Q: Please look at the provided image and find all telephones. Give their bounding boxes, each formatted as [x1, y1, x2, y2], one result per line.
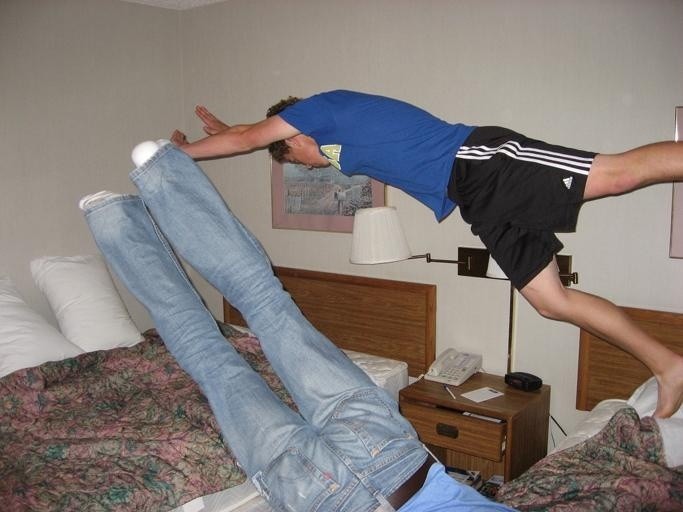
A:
[422, 347, 483, 387]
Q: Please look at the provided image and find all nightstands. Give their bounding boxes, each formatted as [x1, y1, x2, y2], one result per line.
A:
[397, 372, 550, 500]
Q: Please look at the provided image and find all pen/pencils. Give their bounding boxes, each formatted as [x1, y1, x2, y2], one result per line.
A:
[442, 382, 456, 400]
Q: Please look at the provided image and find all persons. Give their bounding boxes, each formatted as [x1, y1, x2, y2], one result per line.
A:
[166, 90, 682, 419]
[76, 135, 525, 511]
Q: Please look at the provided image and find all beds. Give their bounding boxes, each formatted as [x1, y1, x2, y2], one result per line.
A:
[492, 305, 683, 512]
[0, 263, 436, 511]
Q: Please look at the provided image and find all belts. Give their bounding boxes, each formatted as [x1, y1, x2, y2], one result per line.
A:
[374, 454, 435, 511]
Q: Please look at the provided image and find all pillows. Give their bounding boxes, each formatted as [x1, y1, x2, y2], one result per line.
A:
[0, 253, 145, 378]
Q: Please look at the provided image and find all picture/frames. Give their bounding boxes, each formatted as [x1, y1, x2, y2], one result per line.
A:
[268, 151, 387, 234]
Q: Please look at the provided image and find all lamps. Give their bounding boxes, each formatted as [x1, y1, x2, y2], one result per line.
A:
[347, 206, 490, 279]
